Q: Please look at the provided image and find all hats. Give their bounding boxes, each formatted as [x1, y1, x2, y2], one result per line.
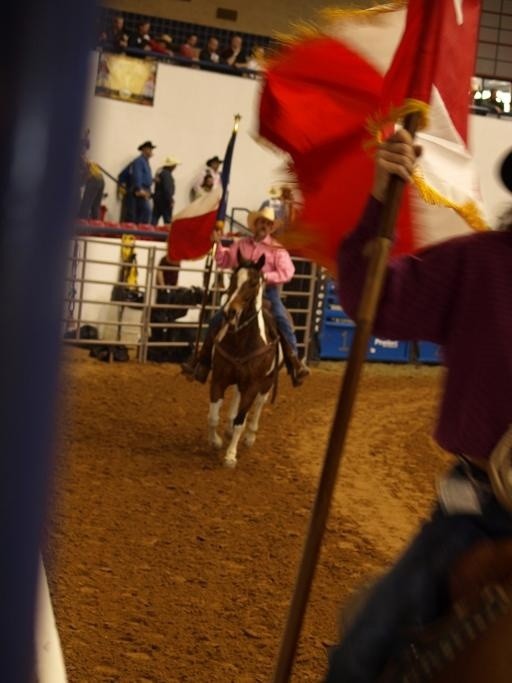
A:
[269, 183, 293, 198]
[248, 207, 283, 233]
[207, 156, 224, 166]
[138, 141, 156, 149]
[163, 156, 182, 167]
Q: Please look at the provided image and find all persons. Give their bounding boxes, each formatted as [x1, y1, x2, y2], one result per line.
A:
[152, 254, 180, 340]
[259, 187, 296, 229]
[480, 89, 504, 114]
[182, 205, 309, 386]
[325, 129, 512, 682]
[117, 142, 179, 226]
[79, 158, 105, 219]
[192, 156, 223, 204]
[99, 14, 268, 80]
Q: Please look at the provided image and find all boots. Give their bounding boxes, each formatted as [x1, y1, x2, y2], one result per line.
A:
[288, 355, 309, 386]
[182, 357, 209, 383]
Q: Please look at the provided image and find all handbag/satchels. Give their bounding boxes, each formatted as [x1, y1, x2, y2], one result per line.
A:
[126, 289, 144, 310]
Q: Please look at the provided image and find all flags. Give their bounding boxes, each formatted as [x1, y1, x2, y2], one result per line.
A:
[258, 0, 501, 272]
[167, 132, 237, 262]
